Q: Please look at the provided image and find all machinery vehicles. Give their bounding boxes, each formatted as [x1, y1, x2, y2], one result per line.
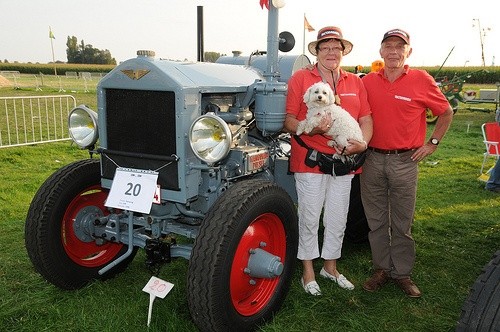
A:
[24, 1, 371, 332]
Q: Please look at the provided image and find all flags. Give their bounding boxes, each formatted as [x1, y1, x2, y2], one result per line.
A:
[50, 31, 55, 39]
[304, 17, 315, 32]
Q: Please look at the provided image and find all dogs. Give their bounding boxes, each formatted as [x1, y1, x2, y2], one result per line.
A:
[296, 81, 364, 149]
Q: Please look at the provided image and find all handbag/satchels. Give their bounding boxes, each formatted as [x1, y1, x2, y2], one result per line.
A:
[292, 130, 366, 176]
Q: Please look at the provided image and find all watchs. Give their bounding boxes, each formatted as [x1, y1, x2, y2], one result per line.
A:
[430, 138, 439, 145]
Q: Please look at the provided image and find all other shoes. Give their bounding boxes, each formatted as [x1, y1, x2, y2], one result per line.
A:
[301, 276, 323, 296]
[320, 267, 354, 291]
[363, 270, 390, 292]
[397, 277, 421, 297]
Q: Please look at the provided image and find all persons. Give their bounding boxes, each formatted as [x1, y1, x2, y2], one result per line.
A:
[359, 29, 454, 298]
[284, 26, 373, 296]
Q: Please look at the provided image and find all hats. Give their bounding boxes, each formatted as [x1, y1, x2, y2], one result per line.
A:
[308, 26, 353, 56]
[382, 29, 409, 46]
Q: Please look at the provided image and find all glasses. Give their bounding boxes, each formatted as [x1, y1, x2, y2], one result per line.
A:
[318, 47, 342, 53]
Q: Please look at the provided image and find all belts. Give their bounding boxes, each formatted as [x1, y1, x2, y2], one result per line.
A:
[368, 146, 408, 156]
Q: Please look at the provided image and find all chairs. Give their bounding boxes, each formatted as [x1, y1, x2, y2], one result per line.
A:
[480, 121, 500, 175]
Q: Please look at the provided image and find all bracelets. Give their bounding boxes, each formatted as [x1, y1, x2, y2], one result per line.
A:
[364, 141, 368, 149]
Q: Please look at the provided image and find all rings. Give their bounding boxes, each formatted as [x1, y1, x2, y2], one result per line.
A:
[327, 125, 330, 128]
